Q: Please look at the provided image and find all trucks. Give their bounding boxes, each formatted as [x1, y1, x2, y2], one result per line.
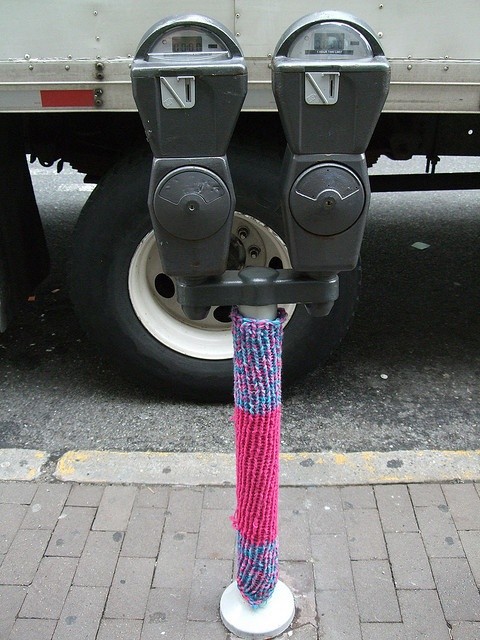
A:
[0, 0, 480, 397]
